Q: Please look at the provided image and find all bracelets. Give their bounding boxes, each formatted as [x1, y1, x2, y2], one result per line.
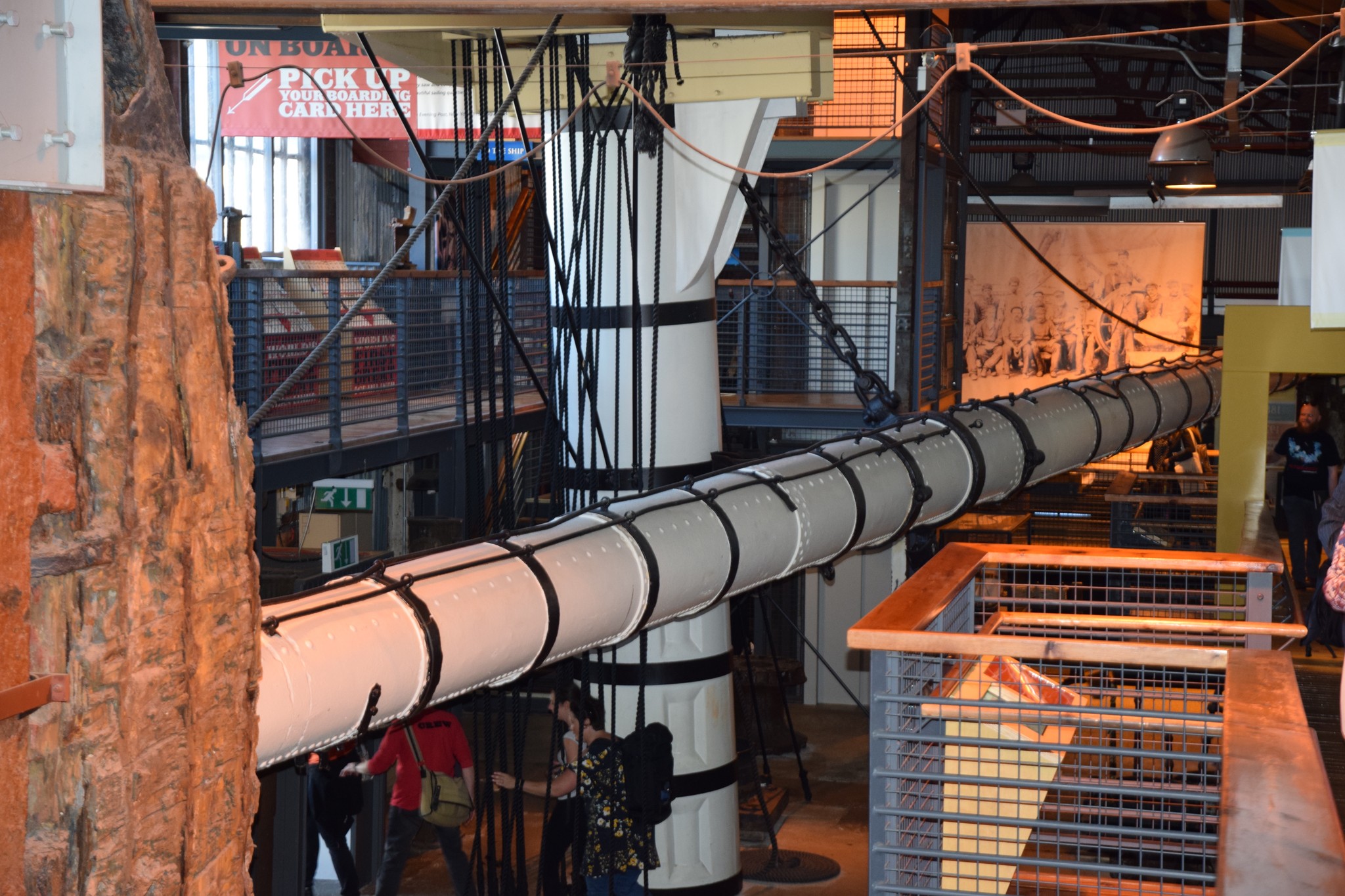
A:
[354, 762, 360, 773]
[516, 778, 526, 794]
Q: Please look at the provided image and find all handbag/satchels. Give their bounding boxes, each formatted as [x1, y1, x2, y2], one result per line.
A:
[420, 766, 474, 828]
[1174, 457, 1207, 495]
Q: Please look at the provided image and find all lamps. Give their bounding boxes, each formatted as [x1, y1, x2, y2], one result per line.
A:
[918, 50, 940, 92]
[995, 100, 1030, 127]
[973, 119, 981, 133]
[1148, 81, 1254, 189]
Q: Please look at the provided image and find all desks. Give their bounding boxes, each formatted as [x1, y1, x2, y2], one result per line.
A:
[939, 513, 1031, 612]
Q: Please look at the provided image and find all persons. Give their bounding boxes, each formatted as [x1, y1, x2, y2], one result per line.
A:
[491, 694, 661, 896]
[1145, 424, 1203, 549]
[294, 741, 364, 896]
[339, 703, 478, 896]
[539, 682, 589, 896]
[963, 248, 1200, 379]
[1266, 402, 1339, 590]
[1319, 468, 1345, 738]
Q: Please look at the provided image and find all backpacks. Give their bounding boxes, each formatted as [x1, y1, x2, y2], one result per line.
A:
[621, 722, 673, 826]
[1323, 521, 1345, 610]
